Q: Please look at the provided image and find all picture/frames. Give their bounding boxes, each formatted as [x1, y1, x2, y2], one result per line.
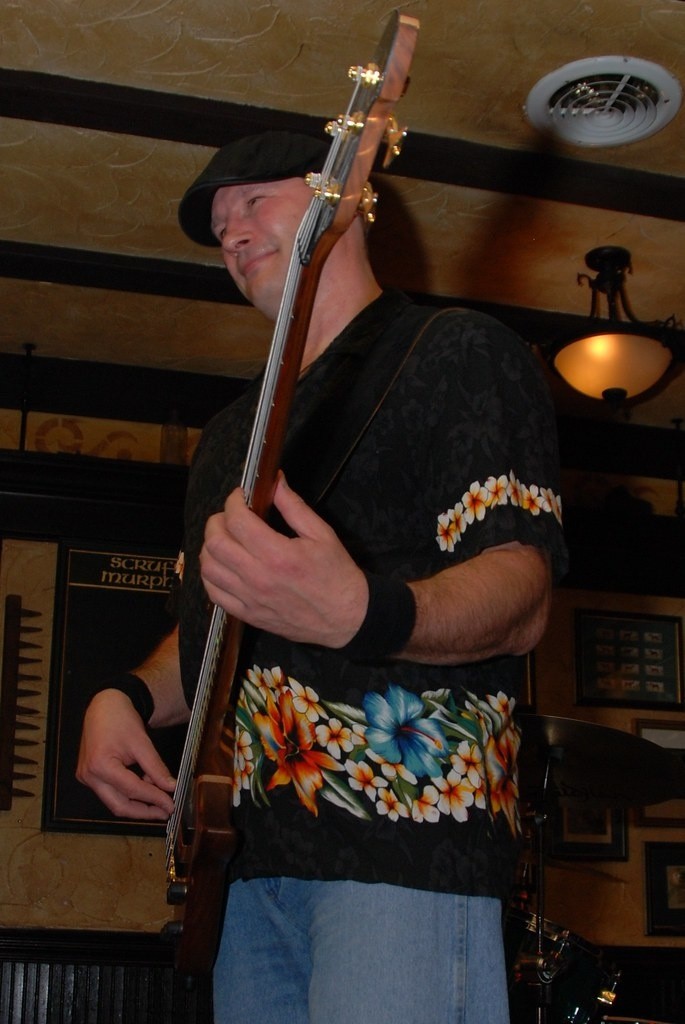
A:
[644, 840, 685, 936]
[633, 716, 685, 828]
[547, 793, 629, 862]
[571, 604, 685, 712]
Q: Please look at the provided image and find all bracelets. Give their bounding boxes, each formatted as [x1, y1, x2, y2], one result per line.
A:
[338, 568, 415, 666]
[79, 674, 156, 717]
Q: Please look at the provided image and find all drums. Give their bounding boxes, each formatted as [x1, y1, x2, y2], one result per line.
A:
[500, 903, 626, 1024]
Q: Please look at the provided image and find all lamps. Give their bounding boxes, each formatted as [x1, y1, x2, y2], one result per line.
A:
[524, 245, 685, 404]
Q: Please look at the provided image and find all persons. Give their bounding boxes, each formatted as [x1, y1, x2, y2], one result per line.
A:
[75, 118, 563, 1024]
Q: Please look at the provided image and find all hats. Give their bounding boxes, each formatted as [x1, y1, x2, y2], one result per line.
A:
[177, 130, 331, 248]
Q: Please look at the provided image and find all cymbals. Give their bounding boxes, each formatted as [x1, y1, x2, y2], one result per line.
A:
[517, 845, 631, 888]
[512, 708, 684, 808]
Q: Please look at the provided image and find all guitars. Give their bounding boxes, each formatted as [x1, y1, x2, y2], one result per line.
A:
[158, 8, 419, 986]
